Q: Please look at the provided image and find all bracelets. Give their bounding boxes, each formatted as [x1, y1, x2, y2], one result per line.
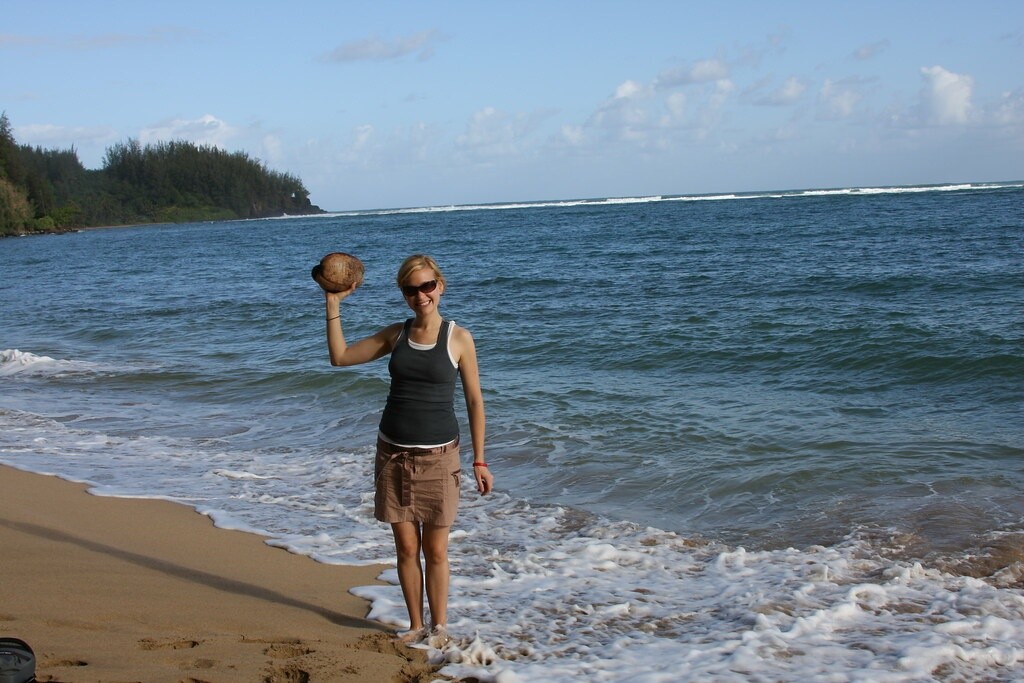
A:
[473, 463, 487, 467]
[326, 315, 341, 321]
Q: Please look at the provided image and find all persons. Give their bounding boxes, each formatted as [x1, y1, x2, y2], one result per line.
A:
[325, 255, 494, 647]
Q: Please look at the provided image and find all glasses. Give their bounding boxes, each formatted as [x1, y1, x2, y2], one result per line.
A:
[401, 279, 439, 298]
